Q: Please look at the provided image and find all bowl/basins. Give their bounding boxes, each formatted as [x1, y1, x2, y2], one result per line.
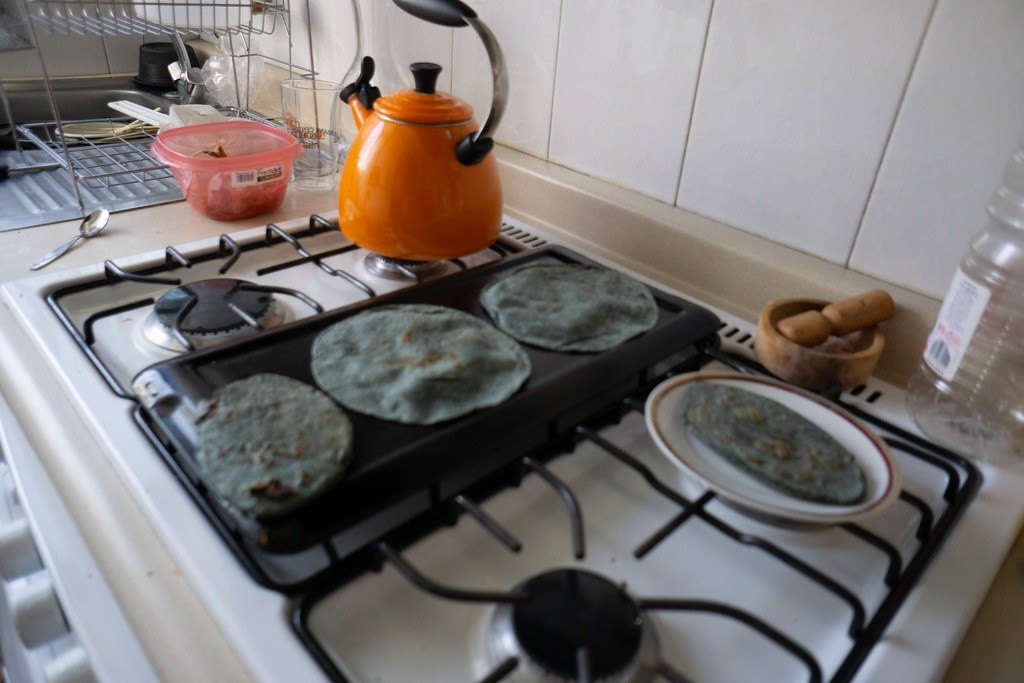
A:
[754, 297, 886, 400]
[150, 120, 305, 222]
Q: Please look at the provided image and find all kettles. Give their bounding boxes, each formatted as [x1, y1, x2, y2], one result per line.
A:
[339, 0, 510, 261]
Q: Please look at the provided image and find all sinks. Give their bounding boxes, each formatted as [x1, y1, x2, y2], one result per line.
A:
[0, 83, 175, 151]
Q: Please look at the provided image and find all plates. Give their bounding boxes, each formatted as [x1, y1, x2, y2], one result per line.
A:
[643, 370, 903, 527]
[54, 122, 128, 138]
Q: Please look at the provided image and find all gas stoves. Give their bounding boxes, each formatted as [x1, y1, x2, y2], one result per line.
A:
[4, 210, 1024, 683]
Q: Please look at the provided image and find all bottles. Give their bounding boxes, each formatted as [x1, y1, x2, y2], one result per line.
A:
[898, 138, 1024, 461]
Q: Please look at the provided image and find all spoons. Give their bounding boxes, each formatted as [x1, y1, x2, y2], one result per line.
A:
[29, 207, 110, 272]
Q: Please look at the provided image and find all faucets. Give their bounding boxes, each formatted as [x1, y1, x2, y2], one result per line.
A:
[167, 33, 205, 104]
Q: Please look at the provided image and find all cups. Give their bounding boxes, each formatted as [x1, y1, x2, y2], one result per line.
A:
[280, 78, 344, 196]
